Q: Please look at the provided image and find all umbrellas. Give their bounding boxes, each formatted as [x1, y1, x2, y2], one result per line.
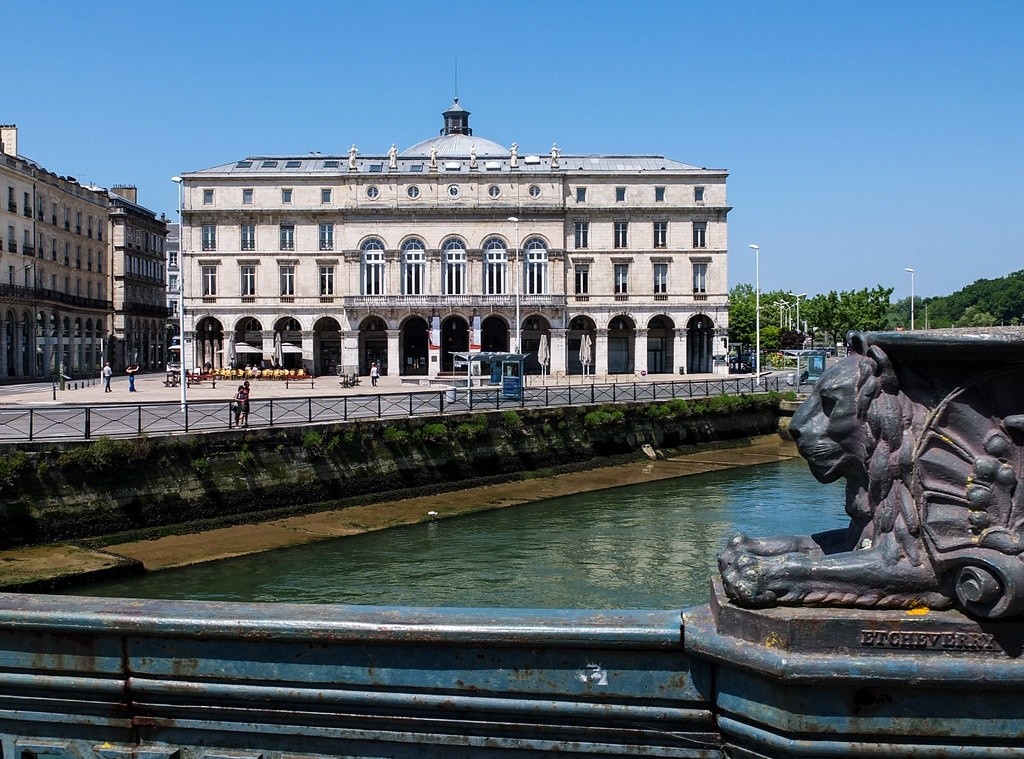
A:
[217, 342, 263, 365]
[272, 333, 284, 368]
[579, 335, 586, 383]
[586, 335, 592, 378]
[270, 343, 307, 353]
[227, 333, 237, 369]
[538, 335, 550, 385]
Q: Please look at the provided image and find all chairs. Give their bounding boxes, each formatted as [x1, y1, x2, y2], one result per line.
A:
[208, 366, 307, 381]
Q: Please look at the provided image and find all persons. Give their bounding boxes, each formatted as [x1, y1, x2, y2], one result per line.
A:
[233, 381, 250, 425]
[371, 362, 379, 387]
[470, 144, 477, 166]
[552, 143, 558, 165]
[103, 362, 112, 392]
[304, 364, 308, 373]
[429, 146, 438, 165]
[347, 144, 358, 168]
[245, 364, 258, 370]
[185, 362, 211, 375]
[126, 364, 139, 392]
[388, 144, 399, 167]
[800, 368, 808, 381]
[510, 143, 519, 165]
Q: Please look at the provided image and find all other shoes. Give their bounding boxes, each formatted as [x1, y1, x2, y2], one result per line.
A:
[241, 425, 248, 428]
[105, 390, 108, 392]
[130, 389, 136, 392]
[109, 390, 111, 392]
[235, 426, 241, 429]
[373, 384, 376, 386]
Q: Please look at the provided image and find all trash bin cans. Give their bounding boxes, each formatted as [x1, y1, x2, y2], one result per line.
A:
[446, 386, 457, 404]
[788, 373, 795, 386]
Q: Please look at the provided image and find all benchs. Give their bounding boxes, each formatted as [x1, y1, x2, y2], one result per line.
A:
[163, 373, 178, 388]
[285, 375, 314, 389]
[187, 375, 216, 389]
[339, 372, 362, 388]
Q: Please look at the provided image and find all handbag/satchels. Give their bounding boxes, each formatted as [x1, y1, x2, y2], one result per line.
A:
[377, 373, 380, 378]
[231, 405, 237, 411]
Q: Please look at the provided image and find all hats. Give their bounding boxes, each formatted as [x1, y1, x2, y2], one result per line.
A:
[254, 364, 257, 366]
